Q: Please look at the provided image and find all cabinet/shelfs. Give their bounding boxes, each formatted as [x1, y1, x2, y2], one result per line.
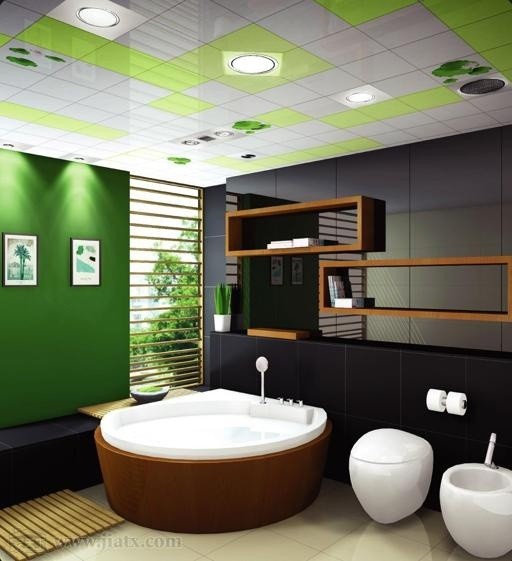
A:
[226, 194, 512, 325]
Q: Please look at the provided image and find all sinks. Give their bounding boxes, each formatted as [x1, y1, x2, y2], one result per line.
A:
[439, 461, 512, 558]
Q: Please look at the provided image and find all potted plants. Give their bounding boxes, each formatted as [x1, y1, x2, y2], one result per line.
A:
[213, 281, 233, 334]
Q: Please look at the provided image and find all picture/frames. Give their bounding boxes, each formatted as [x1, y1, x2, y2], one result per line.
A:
[1, 231, 39, 287]
[69, 236, 103, 287]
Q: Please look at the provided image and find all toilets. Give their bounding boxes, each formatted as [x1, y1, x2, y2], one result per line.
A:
[349, 428, 433, 525]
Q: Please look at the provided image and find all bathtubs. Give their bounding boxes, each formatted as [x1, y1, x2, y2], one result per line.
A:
[93, 387, 332, 534]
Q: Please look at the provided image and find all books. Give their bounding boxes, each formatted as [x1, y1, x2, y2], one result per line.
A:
[266, 238, 319, 249]
[328, 275, 375, 308]
